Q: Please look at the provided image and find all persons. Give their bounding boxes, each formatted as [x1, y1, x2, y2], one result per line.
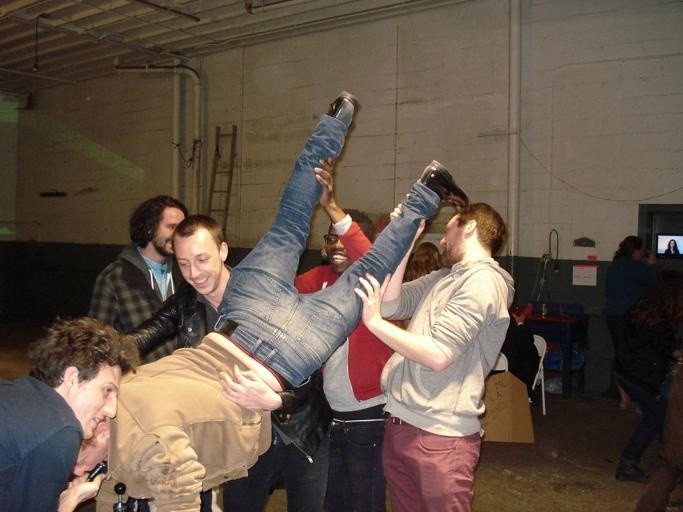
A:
[355, 194, 516, 512]
[297, 212, 400, 512]
[402, 242, 441, 283]
[490, 296, 537, 408]
[612, 272, 683, 484]
[68, 98, 472, 512]
[1, 318, 138, 511]
[665, 239, 679, 254]
[122, 213, 329, 512]
[89, 195, 187, 365]
[605, 235, 655, 412]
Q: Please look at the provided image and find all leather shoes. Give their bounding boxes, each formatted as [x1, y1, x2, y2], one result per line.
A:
[327, 90, 358, 124]
[615, 461, 652, 484]
[417, 160, 470, 210]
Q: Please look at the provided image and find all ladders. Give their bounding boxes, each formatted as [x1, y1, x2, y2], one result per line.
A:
[208, 124, 237, 232]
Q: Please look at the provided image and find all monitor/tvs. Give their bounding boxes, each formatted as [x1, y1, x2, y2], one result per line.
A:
[655, 232, 683, 261]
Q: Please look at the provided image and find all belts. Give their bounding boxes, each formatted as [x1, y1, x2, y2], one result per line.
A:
[392, 417, 406, 425]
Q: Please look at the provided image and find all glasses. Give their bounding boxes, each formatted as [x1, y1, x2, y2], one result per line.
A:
[324, 235, 339, 244]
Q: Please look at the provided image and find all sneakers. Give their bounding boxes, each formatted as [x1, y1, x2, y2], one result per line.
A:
[621, 400, 638, 410]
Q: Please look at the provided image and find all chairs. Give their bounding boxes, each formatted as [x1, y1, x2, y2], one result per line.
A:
[529, 335, 547, 415]
